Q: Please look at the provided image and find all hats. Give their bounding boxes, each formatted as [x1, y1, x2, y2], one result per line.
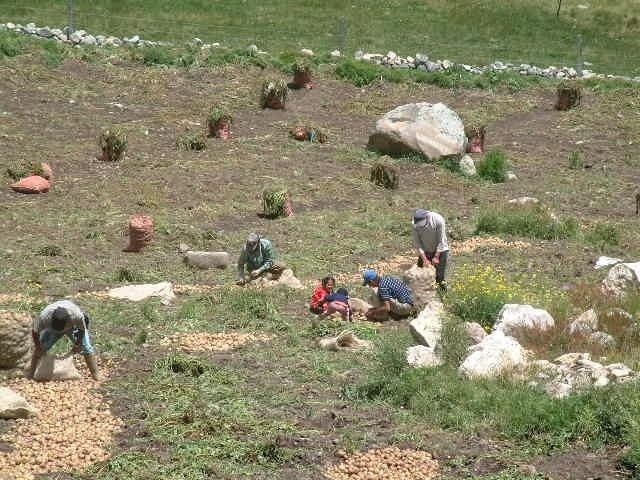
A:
[51, 306, 67, 331]
[247, 233, 259, 252]
[362, 271, 376, 286]
[414, 209, 427, 226]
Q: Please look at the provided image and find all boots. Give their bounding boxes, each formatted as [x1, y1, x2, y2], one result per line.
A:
[87, 357, 104, 380]
[25, 357, 41, 378]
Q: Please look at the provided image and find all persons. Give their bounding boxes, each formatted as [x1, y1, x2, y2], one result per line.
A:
[23, 299, 107, 383]
[411, 208, 450, 294]
[311, 288, 356, 331]
[309, 276, 336, 313]
[361, 270, 418, 322]
[235, 232, 288, 286]
